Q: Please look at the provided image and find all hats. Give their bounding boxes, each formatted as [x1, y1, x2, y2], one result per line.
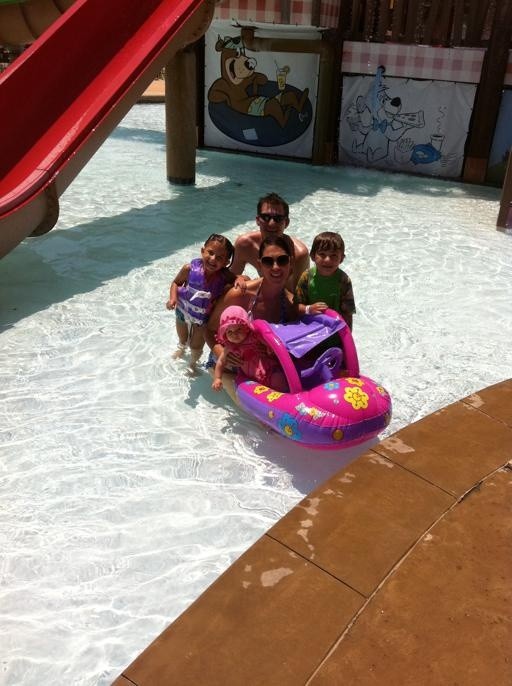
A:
[214, 305, 255, 346]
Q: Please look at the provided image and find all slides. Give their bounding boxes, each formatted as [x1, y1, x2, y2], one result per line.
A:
[1, 2, 214, 254]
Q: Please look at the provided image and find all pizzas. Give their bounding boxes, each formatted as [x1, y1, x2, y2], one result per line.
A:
[392, 111, 425, 128]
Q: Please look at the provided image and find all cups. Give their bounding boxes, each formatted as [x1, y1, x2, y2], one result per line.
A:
[430, 133, 445, 152]
[276, 69, 287, 91]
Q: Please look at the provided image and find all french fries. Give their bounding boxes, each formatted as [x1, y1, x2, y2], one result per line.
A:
[396, 138, 415, 151]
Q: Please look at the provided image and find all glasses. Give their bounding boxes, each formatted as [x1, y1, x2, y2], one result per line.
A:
[258, 213, 287, 223]
[260, 255, 290, 268]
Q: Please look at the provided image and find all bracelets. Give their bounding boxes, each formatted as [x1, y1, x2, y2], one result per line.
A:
[305, 304, 311, 315]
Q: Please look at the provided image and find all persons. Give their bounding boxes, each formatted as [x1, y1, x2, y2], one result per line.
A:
[203, 235, 297, 370]
[211, 305, 287, 392]
[166, 233, 250, 377]
[229, 192, 310, 294]
[291, 232, 356, 332]
